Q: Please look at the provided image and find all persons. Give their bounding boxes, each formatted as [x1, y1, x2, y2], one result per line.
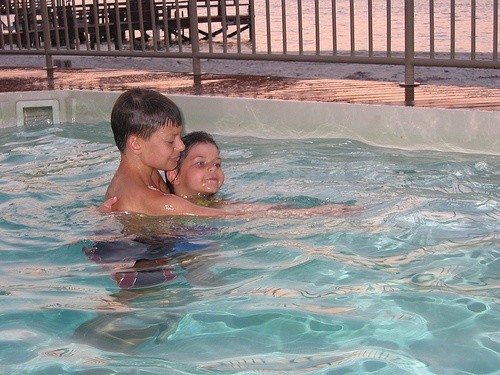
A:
[100, 86, 362, 298]
[90, 129, 297, 287]
